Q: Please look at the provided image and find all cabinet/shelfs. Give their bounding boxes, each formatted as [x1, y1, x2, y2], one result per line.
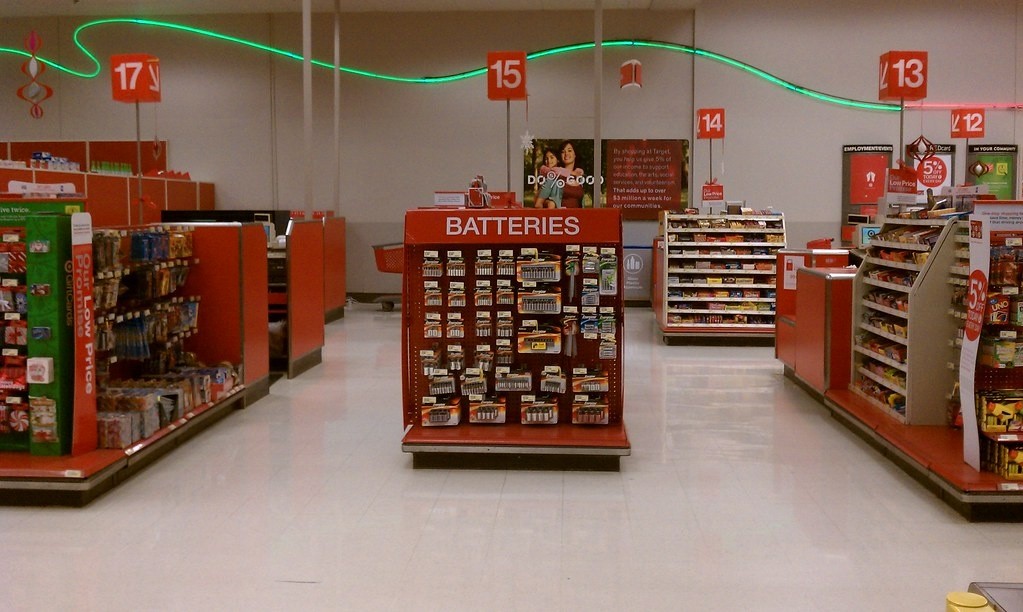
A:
[658, 210, 788, 347]
[848, 193, 1023, 523]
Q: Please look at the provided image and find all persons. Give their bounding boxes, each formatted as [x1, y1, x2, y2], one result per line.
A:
[534, 141, 585, 208]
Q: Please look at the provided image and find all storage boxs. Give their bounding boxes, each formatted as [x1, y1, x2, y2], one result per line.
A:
[869, 267, 896, 279]
[979, 437, 1023, 481]
[877, 271, 900, 282]
[881, 249, 890, 260]
[885, 346, 905, 359]
[941, 211, 974, 219]
[862, 342, 871, 349]
[869, 362, 906, 390]
[888, 227, 939, 245]
[97, 364, 233, 450]
[896, 274, 906, 285]
[896, 295, 907, 311]
[975, 388, 1023, 434]
[890, 250, 906, 263]
[868, 289, 875, 302]
[904, 301, 908, 312]
[894, 323, 907, 339]
[927, 199, 956, 219]
[870, 338, 890, 352]
[897, 205, 928, 219]
[874, 289, 889, 304]
[887, 323, 895, 335]
[878, 344, 899, 355]
[879, 321, 888, 331]
